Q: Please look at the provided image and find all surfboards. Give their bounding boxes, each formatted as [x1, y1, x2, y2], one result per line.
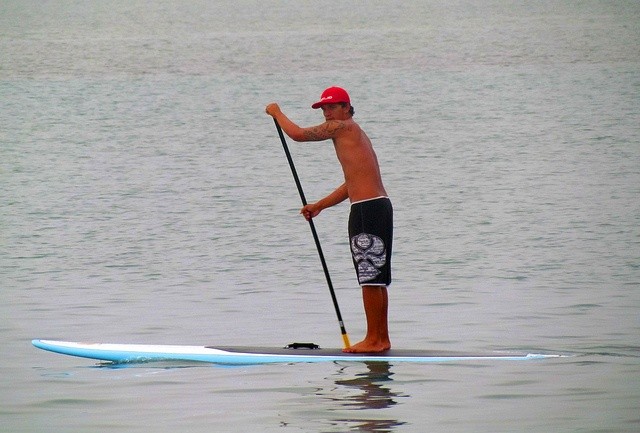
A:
[31, 339, 569, 362]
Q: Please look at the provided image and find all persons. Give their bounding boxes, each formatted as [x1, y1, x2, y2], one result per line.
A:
[265, 86, 394, 353]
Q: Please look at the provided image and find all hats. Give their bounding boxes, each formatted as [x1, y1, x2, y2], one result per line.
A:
[312, 87, 350, 108]
